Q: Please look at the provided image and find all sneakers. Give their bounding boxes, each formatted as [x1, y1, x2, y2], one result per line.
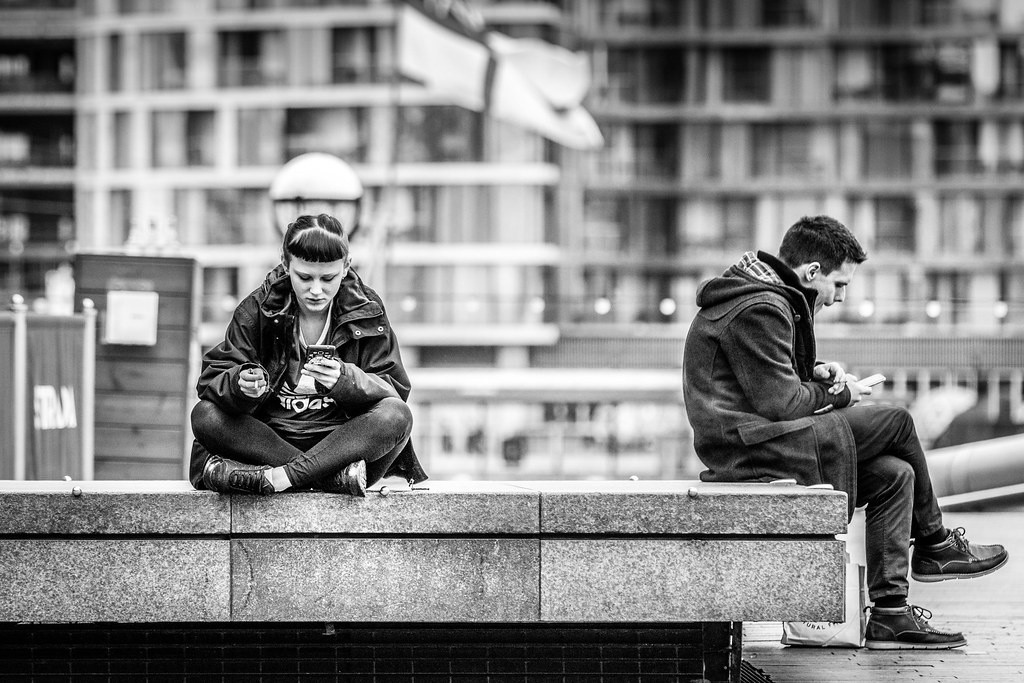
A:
[322, 459, 366, 497]
[202, 455, 275, 496]
[863, 601, 966, 650]
[911, 524, 1008, 581]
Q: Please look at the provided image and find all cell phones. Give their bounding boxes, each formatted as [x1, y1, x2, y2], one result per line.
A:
[855, 374, 886, 387]
[306, 344, 336, 370]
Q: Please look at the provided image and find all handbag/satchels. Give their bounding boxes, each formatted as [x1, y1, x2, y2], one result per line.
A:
[779, 553, 865, 648]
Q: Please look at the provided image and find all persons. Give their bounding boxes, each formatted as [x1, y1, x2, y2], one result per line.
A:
[188, 214, 428, 498]
[681, 214, 1009, 652]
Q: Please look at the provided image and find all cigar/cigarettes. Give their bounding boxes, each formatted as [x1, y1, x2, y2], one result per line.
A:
[253, 380, 259, 389]
[832, 379, 847, 384]
